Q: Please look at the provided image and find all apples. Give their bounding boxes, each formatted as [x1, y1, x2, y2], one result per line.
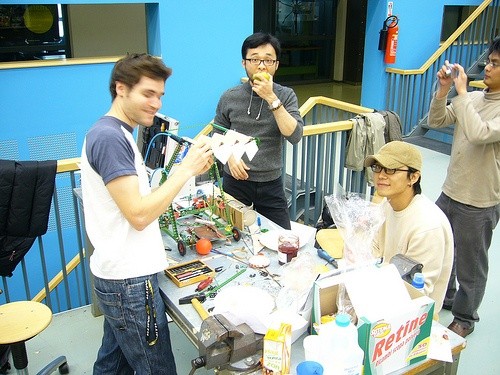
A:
[253, 72, 270, 81]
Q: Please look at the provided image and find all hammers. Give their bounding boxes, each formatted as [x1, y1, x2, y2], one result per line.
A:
[178, 290, 210, 320]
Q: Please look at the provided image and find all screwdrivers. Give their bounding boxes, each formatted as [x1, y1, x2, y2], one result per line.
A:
[317, 248, 338, 268]
[197, 263, 231, 290]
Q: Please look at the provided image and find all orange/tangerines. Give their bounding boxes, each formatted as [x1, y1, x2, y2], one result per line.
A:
[196, 239, 212, 254]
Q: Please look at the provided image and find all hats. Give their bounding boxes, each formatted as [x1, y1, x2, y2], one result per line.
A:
[365, 140, 421, 172]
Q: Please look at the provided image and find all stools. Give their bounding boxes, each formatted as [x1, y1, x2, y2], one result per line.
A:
[0, 301, 69, 375]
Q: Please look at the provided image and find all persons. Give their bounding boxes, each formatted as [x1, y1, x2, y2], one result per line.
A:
[364, 141, 454, 322]
[79, 53, 215, 375]
[211, 33, 304, 230]
[426, 34, 500, 337]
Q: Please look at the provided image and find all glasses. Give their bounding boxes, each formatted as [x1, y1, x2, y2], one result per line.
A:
[245, 57, 277, 66]
[483, 60, 500, 67]
[370, 163, 412, 175]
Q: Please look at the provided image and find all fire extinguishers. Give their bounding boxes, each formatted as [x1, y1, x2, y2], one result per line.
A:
[378, 15, 399, 64]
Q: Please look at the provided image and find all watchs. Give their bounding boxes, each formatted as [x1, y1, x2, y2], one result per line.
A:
[267, 99, 281, 109]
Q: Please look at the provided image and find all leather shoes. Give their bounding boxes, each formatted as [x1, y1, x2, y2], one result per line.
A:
[443, 304, 453, 311]
[447, 321, 475, 338]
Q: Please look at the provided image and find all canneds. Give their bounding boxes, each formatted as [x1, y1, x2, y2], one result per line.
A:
[278, 235, 300, 265]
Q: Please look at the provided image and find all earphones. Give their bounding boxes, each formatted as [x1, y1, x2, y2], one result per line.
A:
[248, 107, 251, 114]
[256, 113, 260, 121]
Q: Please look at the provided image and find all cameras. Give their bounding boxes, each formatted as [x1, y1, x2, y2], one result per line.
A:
[444, 64, 457, 78]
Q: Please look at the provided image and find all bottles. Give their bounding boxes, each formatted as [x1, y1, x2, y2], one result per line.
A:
[296, 361, 323, 375]
[314, 314, 363, 375]
[412, 273, 426, 297]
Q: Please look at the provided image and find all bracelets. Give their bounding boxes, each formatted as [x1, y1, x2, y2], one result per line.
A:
[270, 103, 282, 112]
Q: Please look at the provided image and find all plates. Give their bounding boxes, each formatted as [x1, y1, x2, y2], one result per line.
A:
[213, 286, 275, 324]
[259, 230, 306, 251]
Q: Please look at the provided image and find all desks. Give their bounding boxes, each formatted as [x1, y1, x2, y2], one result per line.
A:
[74, 179, 467, 375]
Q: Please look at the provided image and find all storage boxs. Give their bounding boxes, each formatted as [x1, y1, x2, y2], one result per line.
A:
[309, 268, 436, 375]
